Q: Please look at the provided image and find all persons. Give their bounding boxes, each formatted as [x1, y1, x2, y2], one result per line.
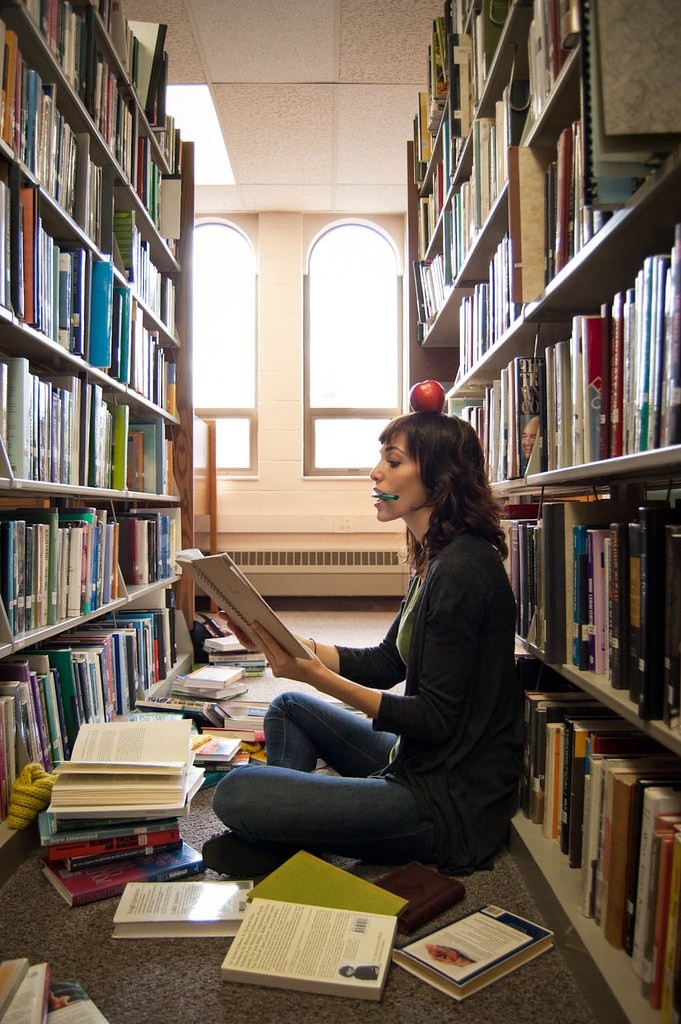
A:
[518, 416, 539, 474]
[337, 963, 381, 982]
[199, 409, 521, 875]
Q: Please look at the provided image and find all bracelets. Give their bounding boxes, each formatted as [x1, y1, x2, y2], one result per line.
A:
[310, 638, 316, 655]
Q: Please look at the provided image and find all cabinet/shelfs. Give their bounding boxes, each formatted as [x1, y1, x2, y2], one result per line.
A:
[403, 0, 681, 1024]
[0, 0, 198, 889]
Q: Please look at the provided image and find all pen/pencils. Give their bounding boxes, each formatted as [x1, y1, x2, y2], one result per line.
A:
[371, 493, 399, 500]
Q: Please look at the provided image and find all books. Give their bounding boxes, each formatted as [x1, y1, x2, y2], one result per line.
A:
[172, 547, 313, 661]
[111, 881, 256, 940]
[0, 1, 320, 913]
[0, 958, 111, 1024]
[371, 861, 466, 937]
[219, 897, 399, 1000]
[392, 903, 555, 1002]
[514, 649, 681, 1024]
[245, 850, 409, 917]
[411, 0, 680, 731]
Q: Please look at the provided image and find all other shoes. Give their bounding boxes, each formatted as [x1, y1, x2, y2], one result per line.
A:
[202, 830, 330, 878]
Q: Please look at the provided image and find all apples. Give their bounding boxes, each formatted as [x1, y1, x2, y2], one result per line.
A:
[409, 379, 446, 413]
